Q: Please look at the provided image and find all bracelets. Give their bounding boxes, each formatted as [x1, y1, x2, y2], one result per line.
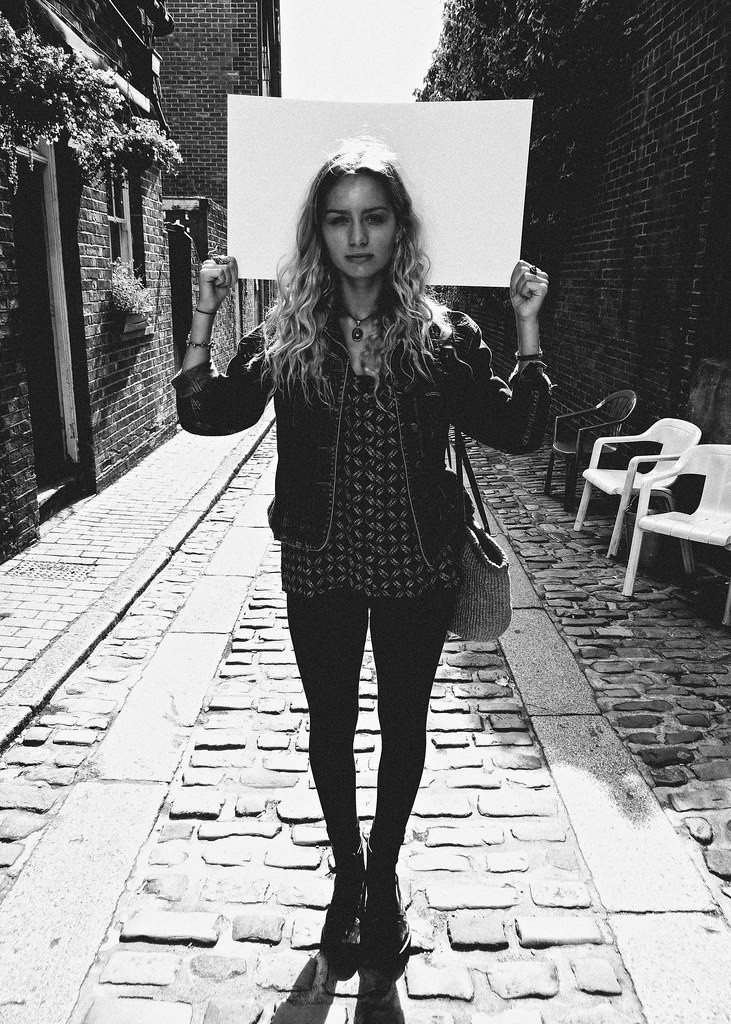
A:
[186, 329, 216, 351]
[196, 304, 215, 316]
[514, 347, 543, 361]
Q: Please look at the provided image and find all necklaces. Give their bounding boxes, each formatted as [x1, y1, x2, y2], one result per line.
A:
[340, 302, 372, 342]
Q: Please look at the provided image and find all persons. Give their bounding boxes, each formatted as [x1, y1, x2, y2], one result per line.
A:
[170, 142, 549, 974]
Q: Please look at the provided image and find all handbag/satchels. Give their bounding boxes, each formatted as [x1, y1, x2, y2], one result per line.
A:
[441, 467, 512, 643]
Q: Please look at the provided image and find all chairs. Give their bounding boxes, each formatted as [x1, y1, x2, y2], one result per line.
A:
[622, 444, 730, 626]
[544, 390, 639, 510]
[573, 418, 704, 559]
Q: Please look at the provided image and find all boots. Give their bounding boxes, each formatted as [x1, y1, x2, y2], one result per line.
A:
[363, 837, 412, 960]
[323, 840, 365, 946]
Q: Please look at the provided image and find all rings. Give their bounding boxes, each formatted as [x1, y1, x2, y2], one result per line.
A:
[529, 264, 538, 275]
